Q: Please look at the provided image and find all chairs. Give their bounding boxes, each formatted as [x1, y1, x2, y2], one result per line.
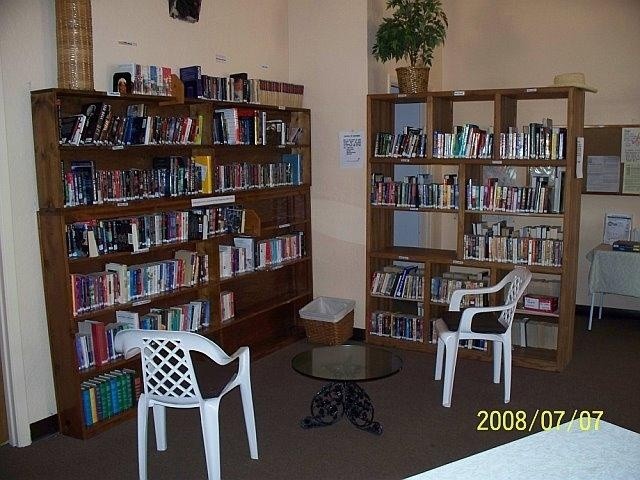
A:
[112, 328, 260, 480]
[433, 267, 532, 409]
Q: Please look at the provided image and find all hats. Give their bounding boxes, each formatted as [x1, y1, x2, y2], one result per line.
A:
[548, 72, 598, 93]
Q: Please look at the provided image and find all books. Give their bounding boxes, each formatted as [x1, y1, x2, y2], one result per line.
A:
[602, 211, 640, 253]
[81, 367, 142, 426]
[219, 230, 305, 278]
[212, 106, 302, 193]
[372, 171, 565, 213]
[107, 62, 304, 109]
[463, 220, 564, 267]
[372, 310, 487, 351]
[220, 291, 234, 321]
[191, 195, 245, 240]
[371, 265, 490, 307]
[374, 118, 567, 159]
[512, 318, 558, 350]
[56, 97, 213, 370]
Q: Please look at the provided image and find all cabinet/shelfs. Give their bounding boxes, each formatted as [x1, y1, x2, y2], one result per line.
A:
[365, 83, 597, 372]
[30, 88, 312, 442]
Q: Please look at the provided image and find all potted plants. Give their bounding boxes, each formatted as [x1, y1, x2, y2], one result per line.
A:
[371, 1, 449, 94]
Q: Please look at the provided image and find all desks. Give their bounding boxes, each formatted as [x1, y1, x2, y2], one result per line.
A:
[585, 242, 640, 331]
[292, 343, 404, 435]
